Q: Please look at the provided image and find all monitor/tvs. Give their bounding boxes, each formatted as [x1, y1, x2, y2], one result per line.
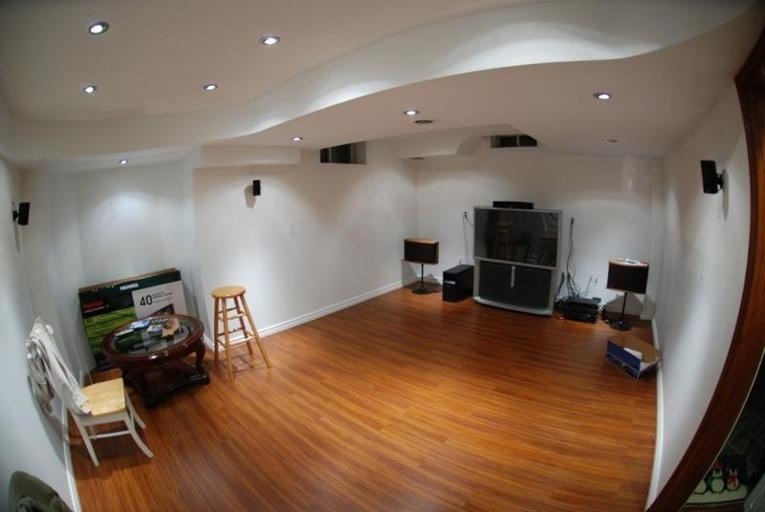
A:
[474, 206, 563, 270]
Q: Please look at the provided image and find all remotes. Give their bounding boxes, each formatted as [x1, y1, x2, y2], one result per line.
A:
[114, 329, 134, 337]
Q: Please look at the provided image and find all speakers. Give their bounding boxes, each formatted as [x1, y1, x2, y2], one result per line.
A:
[253, 180, 260, 195]
[701, 160, 723, 193]
[404, 239, 438, 264]
[14, 202, 30, 225]
[607, 261, 649, 294]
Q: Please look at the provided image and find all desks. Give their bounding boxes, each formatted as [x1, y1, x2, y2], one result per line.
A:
[101, 314, 210, 411]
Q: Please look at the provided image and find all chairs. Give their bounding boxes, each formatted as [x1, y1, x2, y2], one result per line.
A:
[33, 324, 153, 466]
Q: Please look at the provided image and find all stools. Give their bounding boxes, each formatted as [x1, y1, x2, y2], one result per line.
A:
[210, 286, 272, 383]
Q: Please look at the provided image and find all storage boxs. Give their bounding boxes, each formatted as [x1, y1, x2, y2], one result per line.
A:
[605, 330, 662, 382]
[78, 267, 187, 370]
